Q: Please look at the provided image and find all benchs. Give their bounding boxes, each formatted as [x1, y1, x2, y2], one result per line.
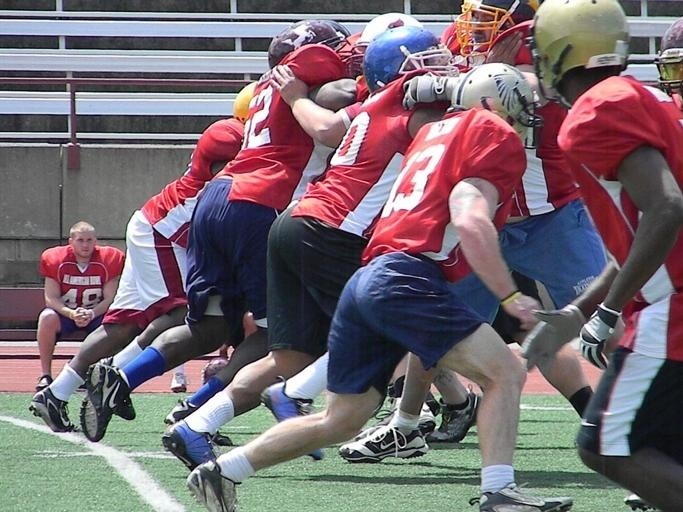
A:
[0, 284, 104, 343]
[0, 1, 683, 142]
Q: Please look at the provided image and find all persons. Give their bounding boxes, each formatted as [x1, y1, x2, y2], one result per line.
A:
[28, 1, 683, 512]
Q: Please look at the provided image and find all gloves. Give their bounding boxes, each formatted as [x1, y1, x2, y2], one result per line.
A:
[578, 303, 624, 371]
[518, 303, 589, 372]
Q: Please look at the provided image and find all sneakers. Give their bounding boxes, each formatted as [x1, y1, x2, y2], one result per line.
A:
[623, 493, 657, 511]
[163, 396, 239, 512]
[337, 374, 483, 465]
[259, 376, 325, 462]
[30, 375, 82, 435]
[169, 371, 189, 391]
[79, 356, 137, 443]
[469, 482, 574, 512]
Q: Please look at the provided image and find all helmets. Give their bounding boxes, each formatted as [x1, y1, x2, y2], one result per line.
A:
[451, 62, 546, 151]
[454, 0, 536, 55]
[354, 12, 462, 94]
[531, 0, 629, 88]
[232, 80, 259, 125]
[268, 17, 353, 69]
[654, 15, 683, 95]
